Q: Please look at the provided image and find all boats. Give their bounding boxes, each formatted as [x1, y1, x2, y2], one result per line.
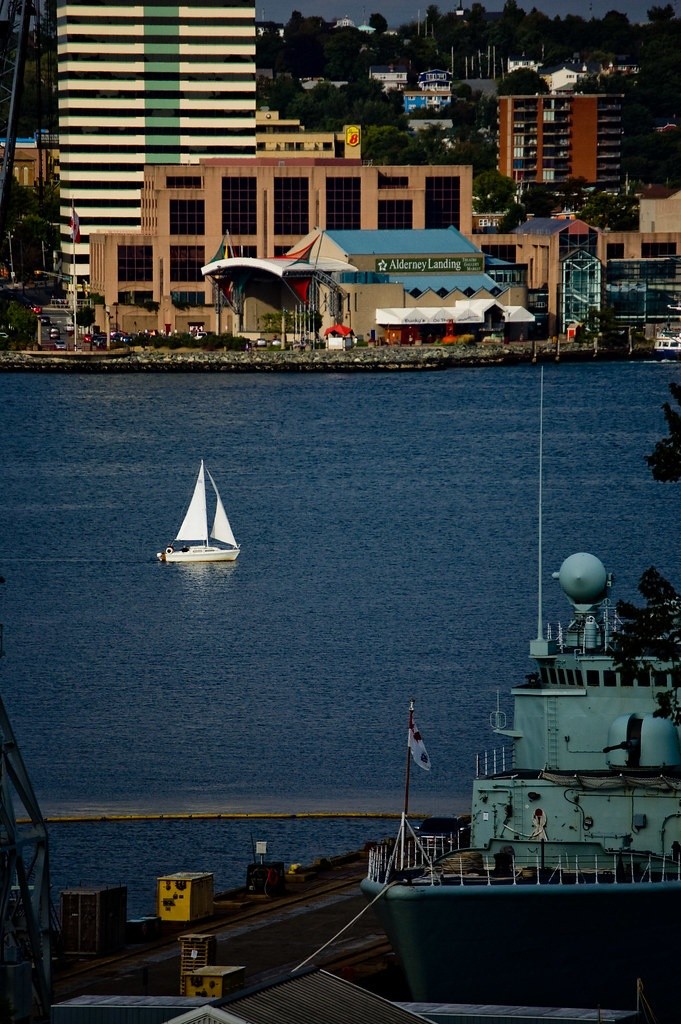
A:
[653, 307, 681, 361]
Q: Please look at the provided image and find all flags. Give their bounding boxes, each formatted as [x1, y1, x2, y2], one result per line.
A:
[408, 716, 431, 771]
[69, 208, 81, 242]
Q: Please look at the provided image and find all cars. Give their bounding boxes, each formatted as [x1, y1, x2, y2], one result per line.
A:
[194, 332, 208, 341]
[32, 304, 133, 350]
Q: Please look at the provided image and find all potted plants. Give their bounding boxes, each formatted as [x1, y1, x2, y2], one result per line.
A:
[205, 337, 216, 351]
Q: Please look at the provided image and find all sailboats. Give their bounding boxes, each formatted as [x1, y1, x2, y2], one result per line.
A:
[156, 457, 241, 563]
